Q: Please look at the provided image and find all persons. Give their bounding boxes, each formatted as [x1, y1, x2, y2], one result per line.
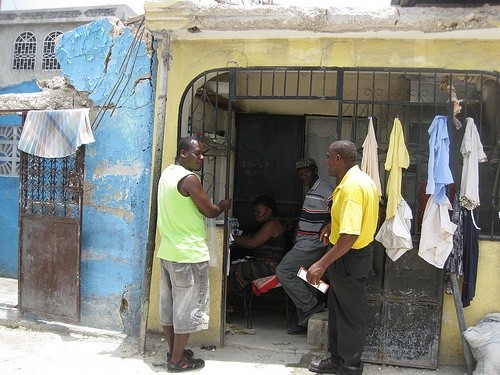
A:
[157, 137, 232, 373]
[224, 198, 288, 324]
[306, 140, 381, 375]
[274, 158, 333, 335]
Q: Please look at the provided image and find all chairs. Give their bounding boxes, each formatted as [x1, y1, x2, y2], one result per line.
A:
[243, 218, 298, 329]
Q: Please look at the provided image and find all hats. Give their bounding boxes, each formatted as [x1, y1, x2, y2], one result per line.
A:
[295, 158, 319, 170]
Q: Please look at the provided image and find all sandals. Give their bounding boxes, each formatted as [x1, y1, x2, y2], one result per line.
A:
[167, 348, 193, 362]
[167, 355, 205, 373]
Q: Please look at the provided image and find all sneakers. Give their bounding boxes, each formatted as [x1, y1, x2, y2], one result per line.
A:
[308, 356, 341, 372]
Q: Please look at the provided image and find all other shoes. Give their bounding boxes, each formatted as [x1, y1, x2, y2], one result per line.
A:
[226, 304, 233, 313]
[299, 302, 326, 325]
[287, 323, 306, 334]
